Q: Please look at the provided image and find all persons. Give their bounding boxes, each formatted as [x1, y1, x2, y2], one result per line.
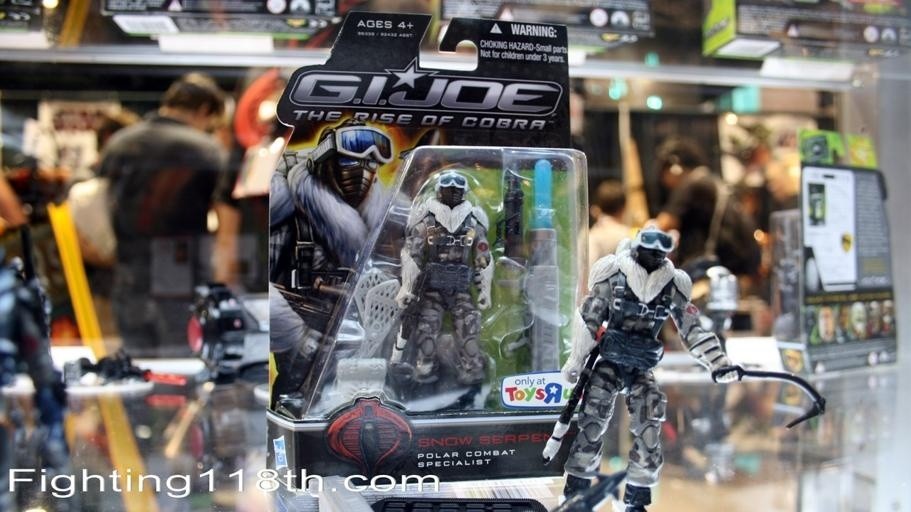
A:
[816, 305, 836, 343]
[1, 64, 291, 471]
[580, 119, 807, 440]
